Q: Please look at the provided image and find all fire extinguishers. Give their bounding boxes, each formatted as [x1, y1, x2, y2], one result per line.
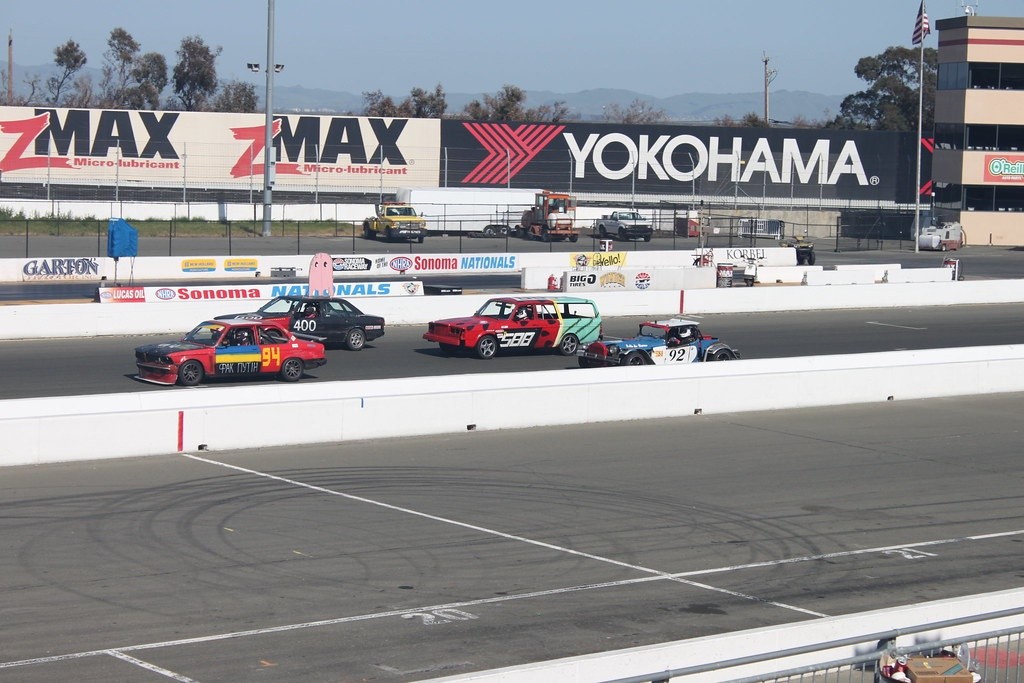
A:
[548, 273, 558, 292]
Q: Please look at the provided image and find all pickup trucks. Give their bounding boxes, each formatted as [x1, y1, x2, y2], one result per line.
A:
[423, 295, 603, 360]
[594, 211, 653, 243]
[362, 202, 426, 245]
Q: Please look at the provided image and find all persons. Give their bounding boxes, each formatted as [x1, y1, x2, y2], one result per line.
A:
[305, 307, 314, 318]
[678, 327, 691, 343]
[517, 308, 529, 320]
[237, 331, 248, 346]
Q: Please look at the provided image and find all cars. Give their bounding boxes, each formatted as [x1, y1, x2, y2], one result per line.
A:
[578, 315, 742, 365]
[211, 294, 386, 351]
[133, 316, 327, 387]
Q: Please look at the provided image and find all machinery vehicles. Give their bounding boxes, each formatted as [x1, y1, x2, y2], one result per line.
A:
[514, 189, 579, 244]
[917, 220, 965, 251]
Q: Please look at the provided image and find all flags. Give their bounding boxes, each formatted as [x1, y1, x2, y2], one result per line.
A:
[912, 0, 930, 45]
